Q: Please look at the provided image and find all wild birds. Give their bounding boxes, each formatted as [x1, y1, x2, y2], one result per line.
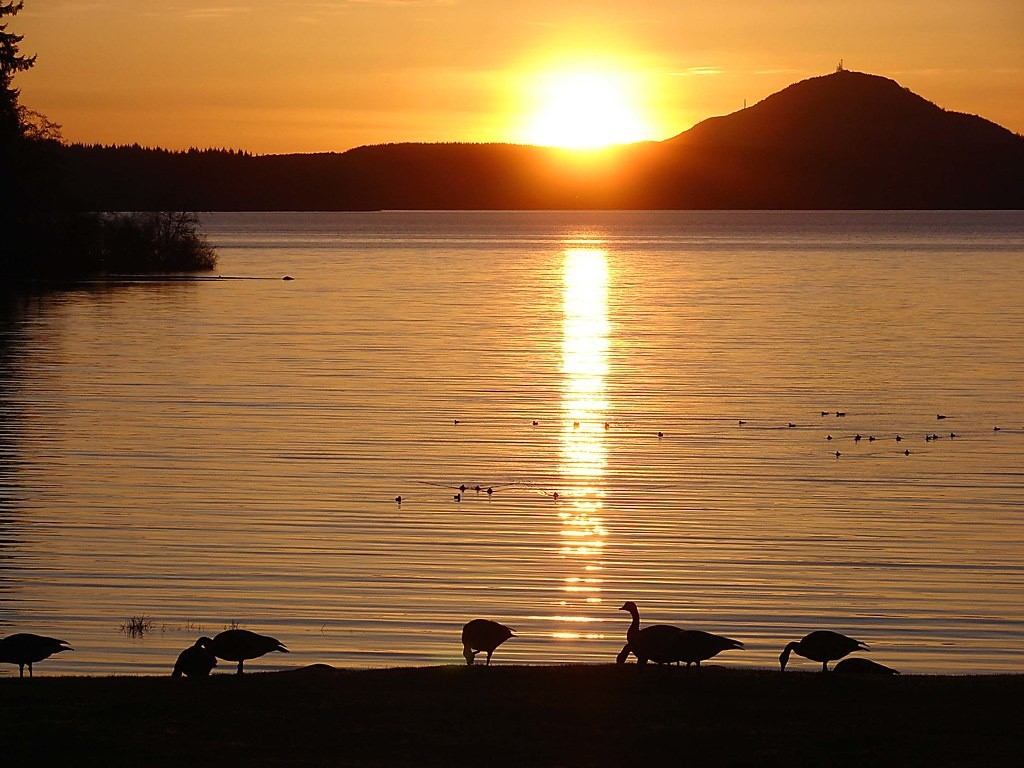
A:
[937, 415, 945, 419]
[896, 436, 901, 439]
[855, 434, 861, 438]
[827, 435, 832, 439]
[533, 421, 538, 425]
[171, 636, 218, 677]
[789, 423, 796, 427]
[837, 412, 845, 416]
[779, 630, 871, 670]
[869, 436, 875, 440]
[574, 422, 579, 425]
[615, 601, 745, 666]
[0, 633, 76, 678]
[739, 421, 746, 424]
[476, 486, 480, 490]
[554, 492, 558, 497]
[487, 488, 492, 494]
[834, 657, 901, 672]
[455, 420, 459, 423]
[933, 434, 938, 439]
[454, 494, 460, 499]
[658, 432, 663, 437]
[605, 422, 609, 427]
[904, 449, 909, 454]
[836, 450, 841, 456]
[461, 618, 517, 666]
[951, 433, 955, 437]
[926, 436, 931, 440]
[994, 426, 1000, 430]
[460, 484, 465, 490]
[822, 412, 829, 415]
[395, 496, 401, 501]
[208, 629, 291, 675]
